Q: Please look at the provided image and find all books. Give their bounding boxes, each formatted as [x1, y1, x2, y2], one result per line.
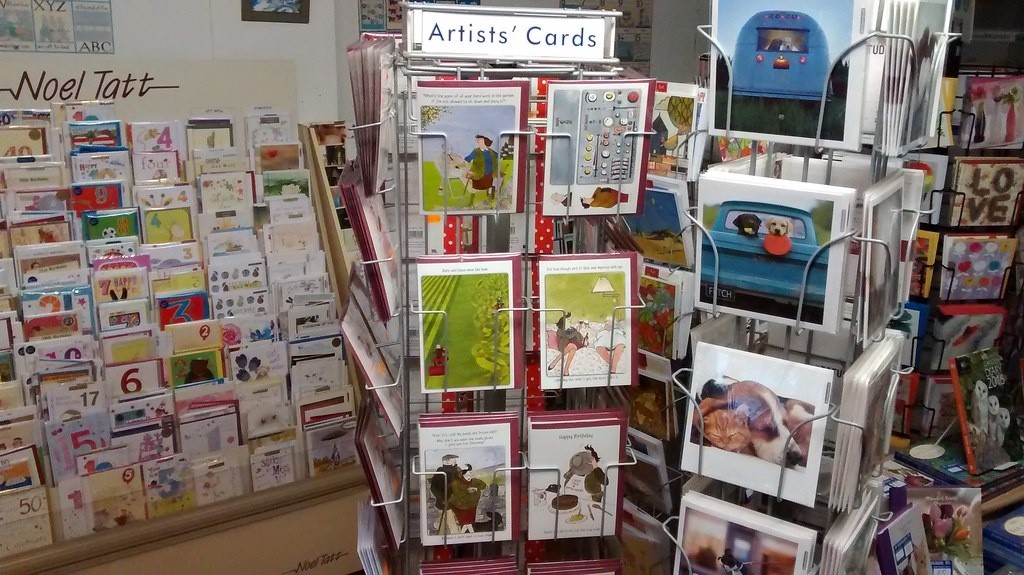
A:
[1, 32, 1024, 575]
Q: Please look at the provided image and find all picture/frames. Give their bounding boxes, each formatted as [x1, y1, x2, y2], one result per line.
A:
[241, 0, 310, 23]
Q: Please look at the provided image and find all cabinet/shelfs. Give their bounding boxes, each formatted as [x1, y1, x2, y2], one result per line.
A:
[0, 0, 1024, 575]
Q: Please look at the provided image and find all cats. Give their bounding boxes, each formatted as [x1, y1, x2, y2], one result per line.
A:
[699, 403, 754, 456]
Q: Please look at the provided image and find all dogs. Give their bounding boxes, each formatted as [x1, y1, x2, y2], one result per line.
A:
[768, 37, 793, 51]
[717, 548, 747, 575]
[694, 380, 815, 469]
[732, 214, 762, 239]
[765, 217, 793, 237]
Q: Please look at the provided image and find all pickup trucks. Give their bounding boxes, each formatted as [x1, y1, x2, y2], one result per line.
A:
[702, 199, 831, 312]
